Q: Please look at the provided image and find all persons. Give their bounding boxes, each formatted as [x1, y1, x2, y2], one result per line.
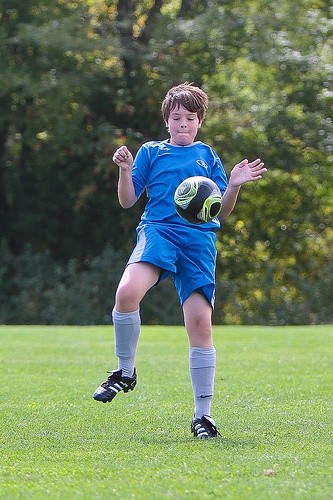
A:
[94, 81, 268, 440]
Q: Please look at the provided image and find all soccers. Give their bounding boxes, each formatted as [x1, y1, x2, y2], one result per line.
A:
[173, 175, 223, 225]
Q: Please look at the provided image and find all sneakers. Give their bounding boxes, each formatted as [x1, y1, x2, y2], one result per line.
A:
[93, 366, 138, 403]
[190, 414, 221, 437]
